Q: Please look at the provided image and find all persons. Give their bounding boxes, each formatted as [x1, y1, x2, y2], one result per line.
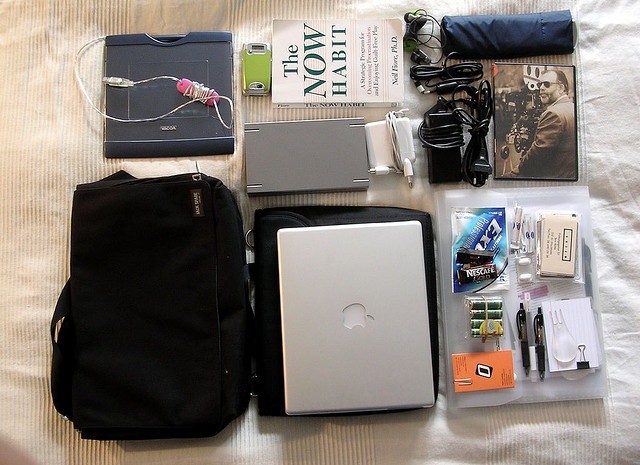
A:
[504, 70, 574, 179]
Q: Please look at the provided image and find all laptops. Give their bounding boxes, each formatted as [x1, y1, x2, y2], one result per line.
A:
[276, 219, 436, 416]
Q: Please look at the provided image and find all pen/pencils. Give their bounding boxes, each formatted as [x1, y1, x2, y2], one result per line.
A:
[516, 303, 530, 377]
[534, 307, 545, 382]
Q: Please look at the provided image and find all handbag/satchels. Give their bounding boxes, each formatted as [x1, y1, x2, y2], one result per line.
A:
[49, 169, 253, 441]
[252, 204, 440, 416]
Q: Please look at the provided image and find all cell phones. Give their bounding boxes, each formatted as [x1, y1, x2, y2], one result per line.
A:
[241, 43, 272, 96]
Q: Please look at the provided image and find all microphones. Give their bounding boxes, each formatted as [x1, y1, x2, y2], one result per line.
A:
[404, 12, 427, 23]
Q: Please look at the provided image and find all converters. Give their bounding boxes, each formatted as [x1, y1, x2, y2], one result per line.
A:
[364, 117, 416, 168]
[424, 112, 462, 184]
